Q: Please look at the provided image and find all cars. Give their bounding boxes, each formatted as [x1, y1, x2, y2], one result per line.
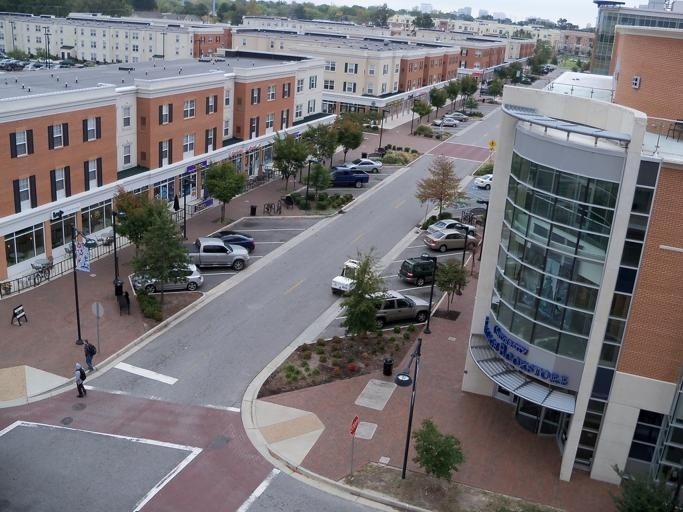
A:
[342, 158, 383, 174]
[516, 62, 555, 85]
[426, 219, 476, 237]
[59, 230, 117, 253]
[31, 258, 51, 269]
[204, 230, 255, 254]
[481, 85, 489, 94]
[423, 229, 479, 252]
[443, 111, 469, 122]
[0, 50, 87, 69]
[473, 173, 492, 190]
[432, 118, 460, 127]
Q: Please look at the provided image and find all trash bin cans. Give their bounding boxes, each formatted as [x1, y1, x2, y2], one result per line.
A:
[362, 153, 367, 158]
[250, 205, 256, 216]
[383, 358, 393, 376]
[115, 280, 123, 296]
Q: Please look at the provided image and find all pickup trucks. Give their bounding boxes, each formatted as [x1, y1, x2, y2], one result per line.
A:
[311, 165, 369, 188]
[174, 237, 250, 271]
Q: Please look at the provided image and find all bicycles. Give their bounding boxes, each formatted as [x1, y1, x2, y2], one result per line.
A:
[32, 267, 51, 285]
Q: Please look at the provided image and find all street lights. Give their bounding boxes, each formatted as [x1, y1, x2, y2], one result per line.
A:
[452, 223, 468, 296]
[409, 97, 421, 135]
[41, 25, 52, 69]
[183, 176, 197, 242]
[70, 221, 98, 346]
[9, 19, 17, 52]
[475, 198, 489, 261]
[480, 68, 488, 97]
[418, 252, 437, 335]
[111, 209, 128, 287]
[305, 158, 319, 208]
[377, 108, 390, 154]
[394, 337, 423, 480]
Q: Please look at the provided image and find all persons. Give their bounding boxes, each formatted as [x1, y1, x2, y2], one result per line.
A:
[74, 363, 86, 398]
[83, 340, 96, 371]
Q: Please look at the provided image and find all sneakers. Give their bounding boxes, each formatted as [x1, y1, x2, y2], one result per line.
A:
[77, 395, 83, 397]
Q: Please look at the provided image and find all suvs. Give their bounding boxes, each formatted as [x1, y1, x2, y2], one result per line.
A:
[133, 262, 205, 292]
[358, 289, 432, 329]
[397, 255, 445, 287]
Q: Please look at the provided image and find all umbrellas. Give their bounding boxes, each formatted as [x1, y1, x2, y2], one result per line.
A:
[173, 193, 179, 211]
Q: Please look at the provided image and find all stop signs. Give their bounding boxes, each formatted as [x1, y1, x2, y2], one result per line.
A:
[350, 414, 359, 435]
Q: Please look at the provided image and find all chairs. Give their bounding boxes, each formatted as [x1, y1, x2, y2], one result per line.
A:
[665, 118, 683, 142]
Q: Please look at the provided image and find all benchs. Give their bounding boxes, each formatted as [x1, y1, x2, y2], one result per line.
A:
[281, 196, 293, 209]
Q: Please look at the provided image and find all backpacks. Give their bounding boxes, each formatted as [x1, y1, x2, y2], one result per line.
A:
[76, 368, 86, 380]
[89, 344, 96, 355]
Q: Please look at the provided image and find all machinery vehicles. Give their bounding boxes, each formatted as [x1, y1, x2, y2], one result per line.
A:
[330, 257, 364, 294]
